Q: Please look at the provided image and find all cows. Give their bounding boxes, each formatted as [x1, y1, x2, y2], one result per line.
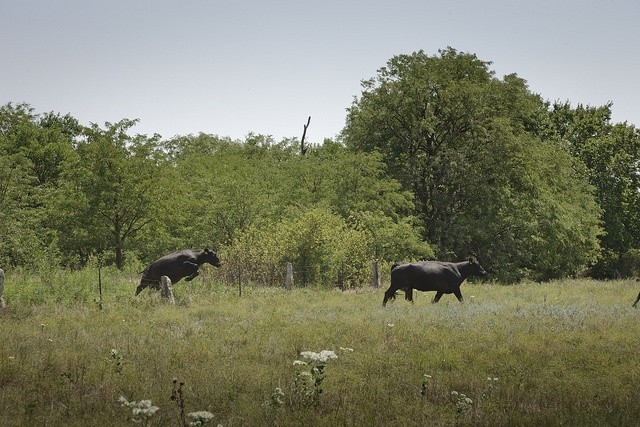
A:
[134, 247, 221, 296]
[382, 255, 487, 308]
